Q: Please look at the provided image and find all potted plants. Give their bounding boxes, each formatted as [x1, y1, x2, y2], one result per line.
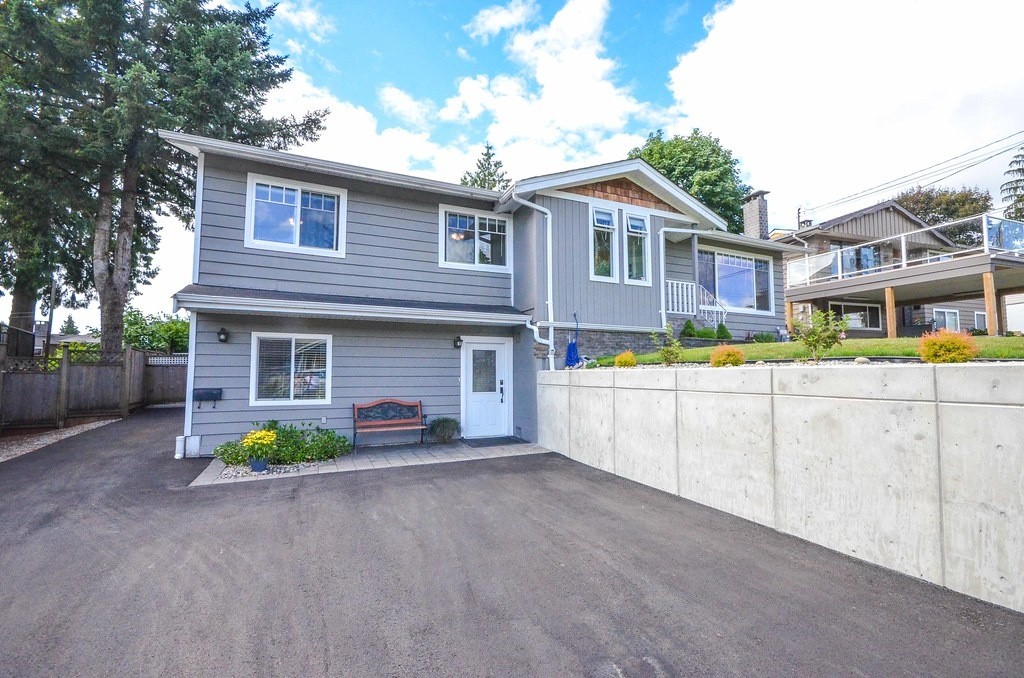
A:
[428, 416, 461, 442]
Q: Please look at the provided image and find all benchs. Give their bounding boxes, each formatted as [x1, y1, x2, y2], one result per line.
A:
[352, 398, 428, 453]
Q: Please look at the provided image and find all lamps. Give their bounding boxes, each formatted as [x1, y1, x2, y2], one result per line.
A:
[454, 335, 463, 347]
[217, 327, 228, 342]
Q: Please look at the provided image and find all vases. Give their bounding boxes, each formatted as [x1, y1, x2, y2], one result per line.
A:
[248, 457, 270, 472]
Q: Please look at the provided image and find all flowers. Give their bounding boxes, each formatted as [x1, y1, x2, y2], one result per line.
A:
[242, 428, 278, 459]
